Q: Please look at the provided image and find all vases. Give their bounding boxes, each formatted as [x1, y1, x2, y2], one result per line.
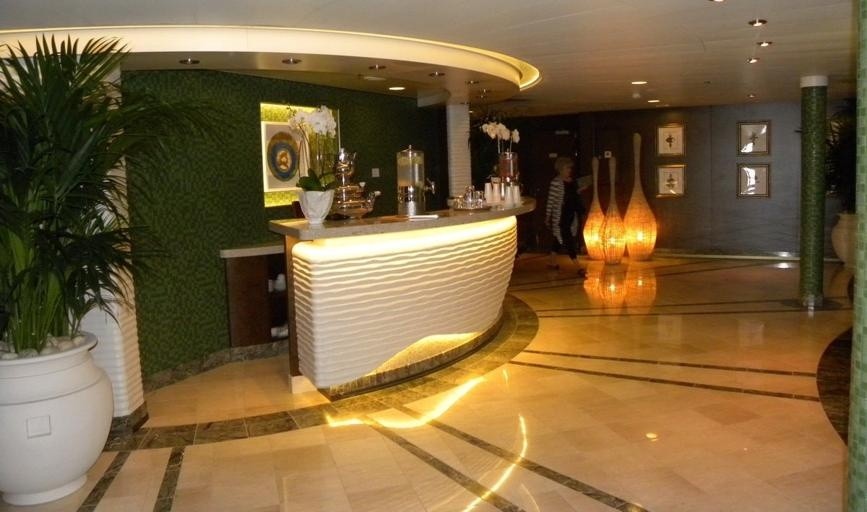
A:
[297, 191, 336, 224]
[500, 176, 521, 200]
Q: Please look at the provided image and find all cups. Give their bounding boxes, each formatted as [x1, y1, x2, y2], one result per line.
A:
[453, 176, 521, 209]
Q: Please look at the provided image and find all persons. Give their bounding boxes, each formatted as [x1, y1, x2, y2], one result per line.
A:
[544, 156, 586, 280]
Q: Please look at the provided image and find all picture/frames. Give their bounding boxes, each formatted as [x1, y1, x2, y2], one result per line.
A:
[738, 121, 770, 198]
[655, 122, 688, 199]
[261, 120, 310, 193]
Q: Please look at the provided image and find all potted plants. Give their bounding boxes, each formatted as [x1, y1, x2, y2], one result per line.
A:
[1, 34, 251, 505]
[795, 88, 857, 269]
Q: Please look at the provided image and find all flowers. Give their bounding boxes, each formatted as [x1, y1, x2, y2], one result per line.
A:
[466, 102, 521, 182]
[286, 103, 342, 190]
